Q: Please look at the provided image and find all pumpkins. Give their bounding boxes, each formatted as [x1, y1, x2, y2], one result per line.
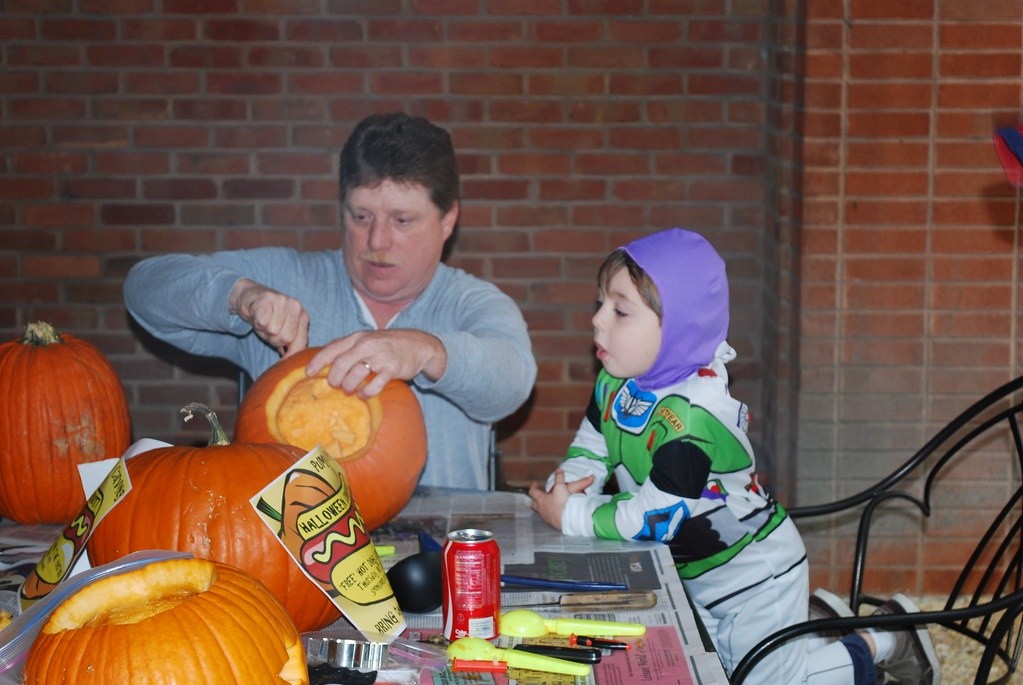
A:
[0, 320, 131, 524]
[84, 400, 346, 634]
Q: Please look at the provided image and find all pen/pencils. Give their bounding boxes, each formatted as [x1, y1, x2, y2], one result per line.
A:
[500, 573, 629, 593]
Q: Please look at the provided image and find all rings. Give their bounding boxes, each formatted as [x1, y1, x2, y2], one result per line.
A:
[359, 361, 372, 375]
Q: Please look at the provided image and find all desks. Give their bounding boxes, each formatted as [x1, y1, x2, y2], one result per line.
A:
[0, 485, 731, 685]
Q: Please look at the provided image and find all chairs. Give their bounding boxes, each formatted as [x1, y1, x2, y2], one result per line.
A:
[729, 377, 1023, 685]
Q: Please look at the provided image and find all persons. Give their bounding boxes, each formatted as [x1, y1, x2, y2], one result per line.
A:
[120, 112, 540, 491]
[528, 226, 940, 685]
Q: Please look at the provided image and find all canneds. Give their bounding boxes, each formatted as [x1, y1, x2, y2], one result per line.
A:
[440, 528, 502, 644]
